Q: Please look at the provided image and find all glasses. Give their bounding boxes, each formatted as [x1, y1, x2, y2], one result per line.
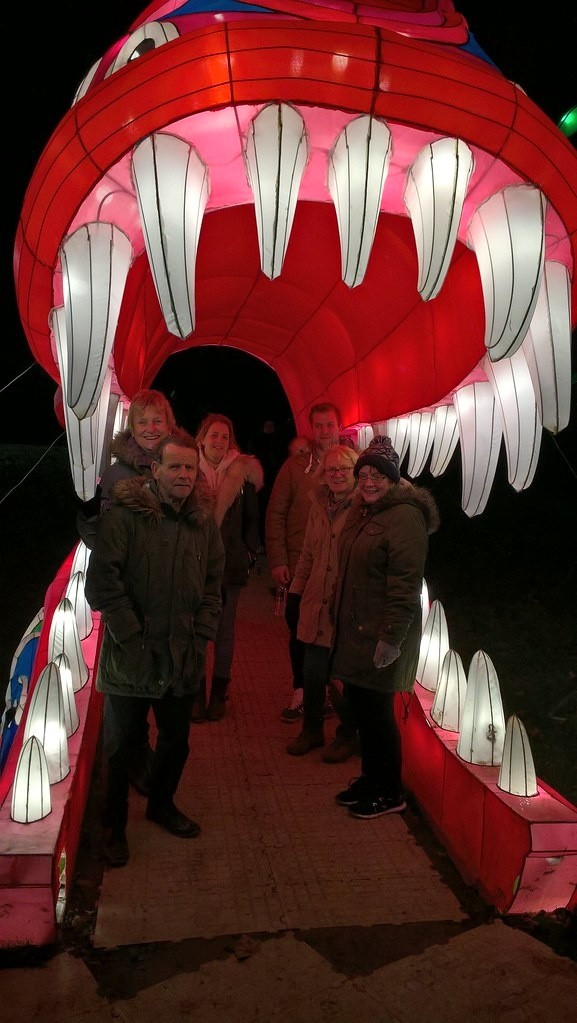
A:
[356, 472, 386, 482]
[324, 465, 353, 477]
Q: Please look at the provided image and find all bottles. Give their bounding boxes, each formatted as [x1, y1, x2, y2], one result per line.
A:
[275, 580, 287, 616]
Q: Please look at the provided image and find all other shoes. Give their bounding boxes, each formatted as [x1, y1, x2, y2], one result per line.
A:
[147, 801, 200, 837]
[102, 829, 129, 867]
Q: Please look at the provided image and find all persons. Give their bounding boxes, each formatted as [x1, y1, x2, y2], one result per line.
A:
[262, 403, 440, 818]
[73, 388, 263, 796]
[84, 429, 234, 862]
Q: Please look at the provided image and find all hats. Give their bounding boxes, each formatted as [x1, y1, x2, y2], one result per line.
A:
[354, 435, 401, 482]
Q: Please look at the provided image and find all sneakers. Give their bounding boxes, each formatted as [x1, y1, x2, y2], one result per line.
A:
[280, 688, 303, 722]
[350, 793, 408, 819]
[335, 775, 375, 805]
[322, 737, 360, 763]
[286, 730, 324, 755]
[325, 686, 335, 717]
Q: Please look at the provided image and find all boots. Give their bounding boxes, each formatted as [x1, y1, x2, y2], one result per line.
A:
[193, 692, 205, 720]
[206, 674, 230, 720]
